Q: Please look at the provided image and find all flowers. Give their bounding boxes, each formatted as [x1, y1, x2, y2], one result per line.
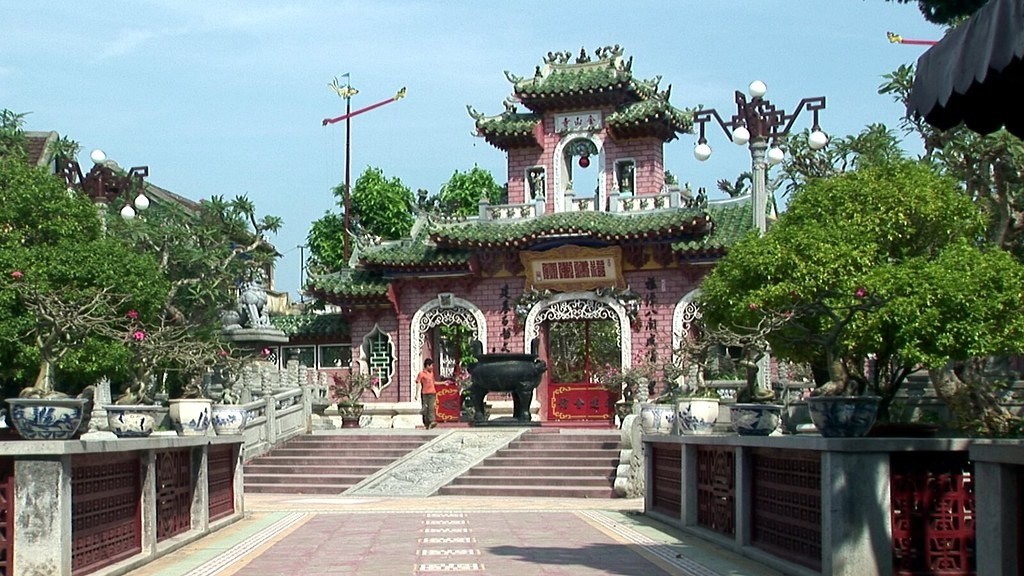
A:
[682, 300, 795, 406]
[596, 362, 656, 403]
[8, 270, 271, 399]
[323, 370, 382, 405]
[802, 288, 901, 396]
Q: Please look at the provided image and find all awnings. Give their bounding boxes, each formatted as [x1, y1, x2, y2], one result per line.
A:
[907, 0, 1024, 140]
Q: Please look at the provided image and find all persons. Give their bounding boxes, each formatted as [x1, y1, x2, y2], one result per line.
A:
[531, 170, 543, 196]
[415, 359, 449, 430]
[620, 163, 632, 192]
[583, 362, 621, 382]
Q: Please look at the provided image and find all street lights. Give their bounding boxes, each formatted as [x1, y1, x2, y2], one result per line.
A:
[694, 79, 828, 400]
[53, 147, 153, 248]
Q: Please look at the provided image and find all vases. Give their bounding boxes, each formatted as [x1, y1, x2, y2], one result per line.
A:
[215, 404, 245, 434]
[337, 403, 364, 428]
[726, 402, 783, 434]
[101, 405, 164, 437]
[2, 398, 90, 439]
[614, 402, 638, 430]
[804, 392, 886, 435]
[168, 397, 214, 435]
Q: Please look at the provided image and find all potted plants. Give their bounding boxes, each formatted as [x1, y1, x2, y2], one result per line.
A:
[460, 376, 492, 423]
[639, 325, 720, 434]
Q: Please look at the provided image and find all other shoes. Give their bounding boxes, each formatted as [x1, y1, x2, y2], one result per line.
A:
[429, 422, 437, 429]
[425, 425, 429, 429]
